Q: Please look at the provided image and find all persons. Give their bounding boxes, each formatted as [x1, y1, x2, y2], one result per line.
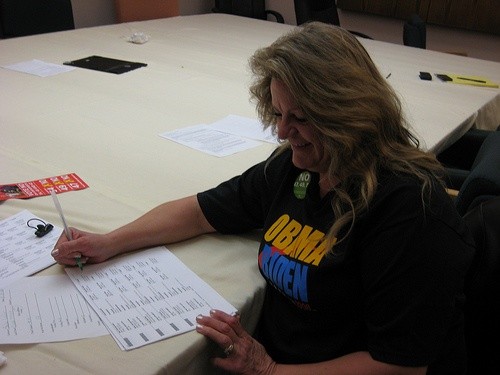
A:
[50, 20, 476, 375]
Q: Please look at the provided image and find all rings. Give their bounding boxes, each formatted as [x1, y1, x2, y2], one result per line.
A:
[225, 344, 233, 353]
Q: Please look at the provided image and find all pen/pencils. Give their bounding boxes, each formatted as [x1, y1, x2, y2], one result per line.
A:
[50, 187, 83, 271]
[456, 77, 486, 83]
[385, 72, 391, 79]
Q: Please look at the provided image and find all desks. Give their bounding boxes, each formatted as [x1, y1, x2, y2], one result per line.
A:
[0, 13, 500, 375]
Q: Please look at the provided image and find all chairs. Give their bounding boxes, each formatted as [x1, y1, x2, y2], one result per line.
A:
[294, 0, 373, 40]
[432, 121, 500, 375]
[212, 0, 285, 24]
